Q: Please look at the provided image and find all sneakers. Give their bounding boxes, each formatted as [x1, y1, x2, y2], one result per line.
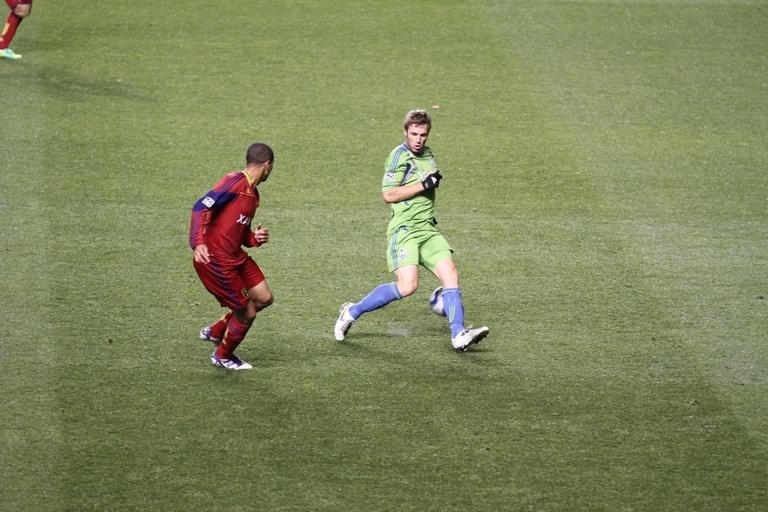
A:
[199, 326, 221, 342]
[451, 325, 489, 352]
[334, 302, 356, 342]
[212, 351, 254, 372]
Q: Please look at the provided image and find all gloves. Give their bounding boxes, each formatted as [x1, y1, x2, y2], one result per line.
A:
[422, 170, 443, 191]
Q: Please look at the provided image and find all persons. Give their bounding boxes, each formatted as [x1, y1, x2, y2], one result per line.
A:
[335, 110, 489, 351]
[0, 0, 33, 59]
[190, 143, 274, 370]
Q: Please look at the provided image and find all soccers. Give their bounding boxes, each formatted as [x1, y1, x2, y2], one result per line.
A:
[429, 285, 448, 315]
